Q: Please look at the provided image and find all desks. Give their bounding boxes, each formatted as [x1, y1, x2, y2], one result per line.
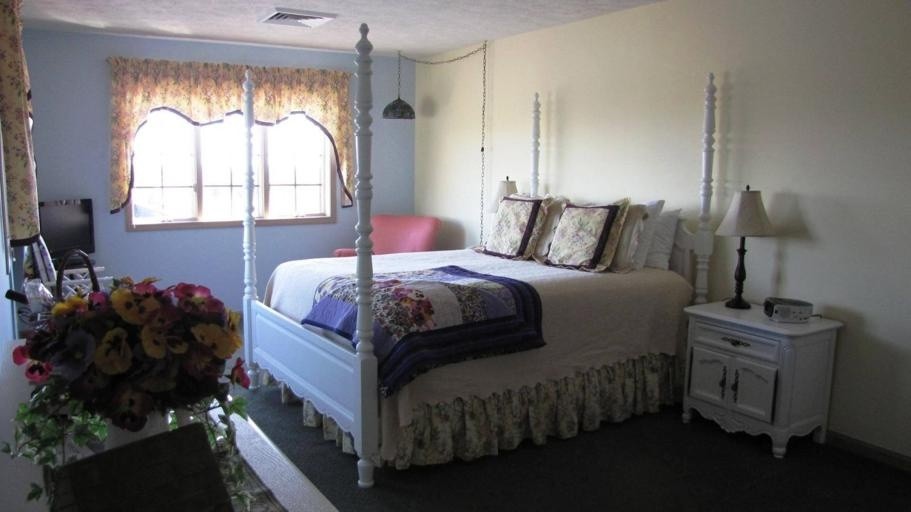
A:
[0, 393, 341, 512]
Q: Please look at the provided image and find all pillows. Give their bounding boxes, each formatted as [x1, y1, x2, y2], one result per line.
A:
[484, 191, 683, 275]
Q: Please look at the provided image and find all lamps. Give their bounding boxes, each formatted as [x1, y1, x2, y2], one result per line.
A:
[380, 38, 495, 246]
[712, 182, 778, 311]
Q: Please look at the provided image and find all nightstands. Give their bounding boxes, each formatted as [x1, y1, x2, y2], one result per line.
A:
[680, 298, 846, 468]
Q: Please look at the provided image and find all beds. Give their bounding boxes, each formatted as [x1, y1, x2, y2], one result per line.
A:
[238, 19, 722, 489]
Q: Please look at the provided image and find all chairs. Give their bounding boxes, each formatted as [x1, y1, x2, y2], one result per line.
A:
[31, 231, 115, 301]
[329, 213, 443, 259]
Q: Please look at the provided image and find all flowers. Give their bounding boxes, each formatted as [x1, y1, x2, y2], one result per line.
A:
[11, 274, 252, 437]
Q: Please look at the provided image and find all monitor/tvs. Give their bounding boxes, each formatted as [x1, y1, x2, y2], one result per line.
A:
[38, 198, 95, 269]
